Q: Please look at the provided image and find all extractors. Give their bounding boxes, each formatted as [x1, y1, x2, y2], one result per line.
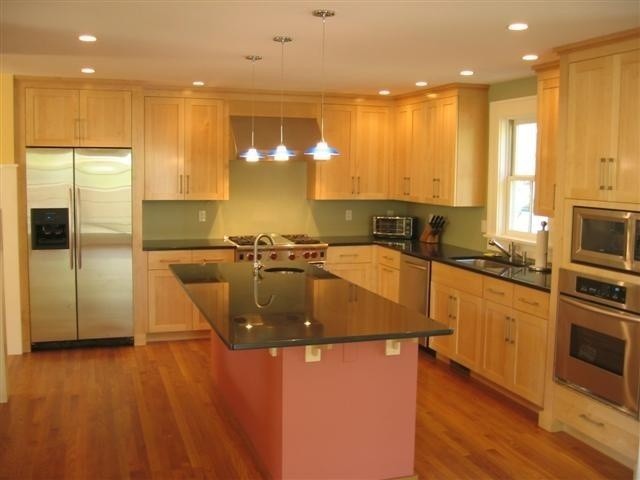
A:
[231, 116, 340, 161]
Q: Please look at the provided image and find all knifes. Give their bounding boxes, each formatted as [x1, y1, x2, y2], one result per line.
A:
[429, 215, 446, 229]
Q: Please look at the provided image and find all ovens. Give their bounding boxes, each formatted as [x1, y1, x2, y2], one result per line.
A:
[371, 216, 416, 240]
[555, 204, 640, 420]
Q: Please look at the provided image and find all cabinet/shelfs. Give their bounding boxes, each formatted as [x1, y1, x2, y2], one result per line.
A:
[320, 246, 372, 329]
[149, 254, 229, 333]
[558, 62, 638, 204]
[321, 99, 396, 195]
[139, 98, 225, 201]
[535, 69, 560, 211]
[429, 262, 482, 364]
[26, 92, 136, 143]
[378, 248, 426, 344]
[483, 267, 554, 412]
[400, 98, 458, 206]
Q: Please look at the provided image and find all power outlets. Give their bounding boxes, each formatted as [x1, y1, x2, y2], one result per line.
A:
[197, 209, 209, 224]
[345, 212, 353, 223]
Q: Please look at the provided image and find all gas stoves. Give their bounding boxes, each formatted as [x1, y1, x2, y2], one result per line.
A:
[225, 233, 329, 263]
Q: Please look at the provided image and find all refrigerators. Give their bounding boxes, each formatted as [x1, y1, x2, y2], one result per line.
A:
[26, 147, 134, 350]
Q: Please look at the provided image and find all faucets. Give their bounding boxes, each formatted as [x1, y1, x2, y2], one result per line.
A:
[252, 232, 276, 270]
[252, 272, 277, 310]
[488, 238, 512, 257]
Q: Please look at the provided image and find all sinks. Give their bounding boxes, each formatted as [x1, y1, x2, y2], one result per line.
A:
[448, 254, 516, 269]
[264, 267, 303, 275]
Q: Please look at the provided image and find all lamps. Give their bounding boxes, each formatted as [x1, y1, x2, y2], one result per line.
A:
[268, 35, 296, 161]
[241, 55, 266, 164]
[304, 9, 342, 160]
